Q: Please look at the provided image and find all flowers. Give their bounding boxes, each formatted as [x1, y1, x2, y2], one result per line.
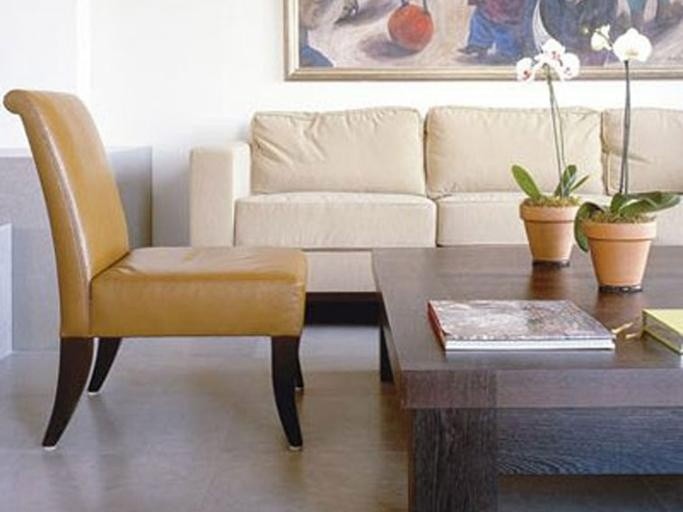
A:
[510, 23, 683, 252]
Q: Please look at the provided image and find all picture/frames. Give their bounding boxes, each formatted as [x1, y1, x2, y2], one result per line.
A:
[279, 1, 683, 84]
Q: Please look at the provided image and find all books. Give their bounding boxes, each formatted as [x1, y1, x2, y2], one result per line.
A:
[427, 296, 618, 353]
[639, 306, 682, 355]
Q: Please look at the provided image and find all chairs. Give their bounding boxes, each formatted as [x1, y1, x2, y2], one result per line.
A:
[0, 85, 314, 456]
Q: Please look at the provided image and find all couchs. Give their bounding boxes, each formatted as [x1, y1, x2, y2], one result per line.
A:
[182, 106, 683, 318]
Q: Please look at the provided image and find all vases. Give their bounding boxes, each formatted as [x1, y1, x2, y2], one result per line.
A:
[580, 219, 657, 292]
[516, 200, 578, 267]
